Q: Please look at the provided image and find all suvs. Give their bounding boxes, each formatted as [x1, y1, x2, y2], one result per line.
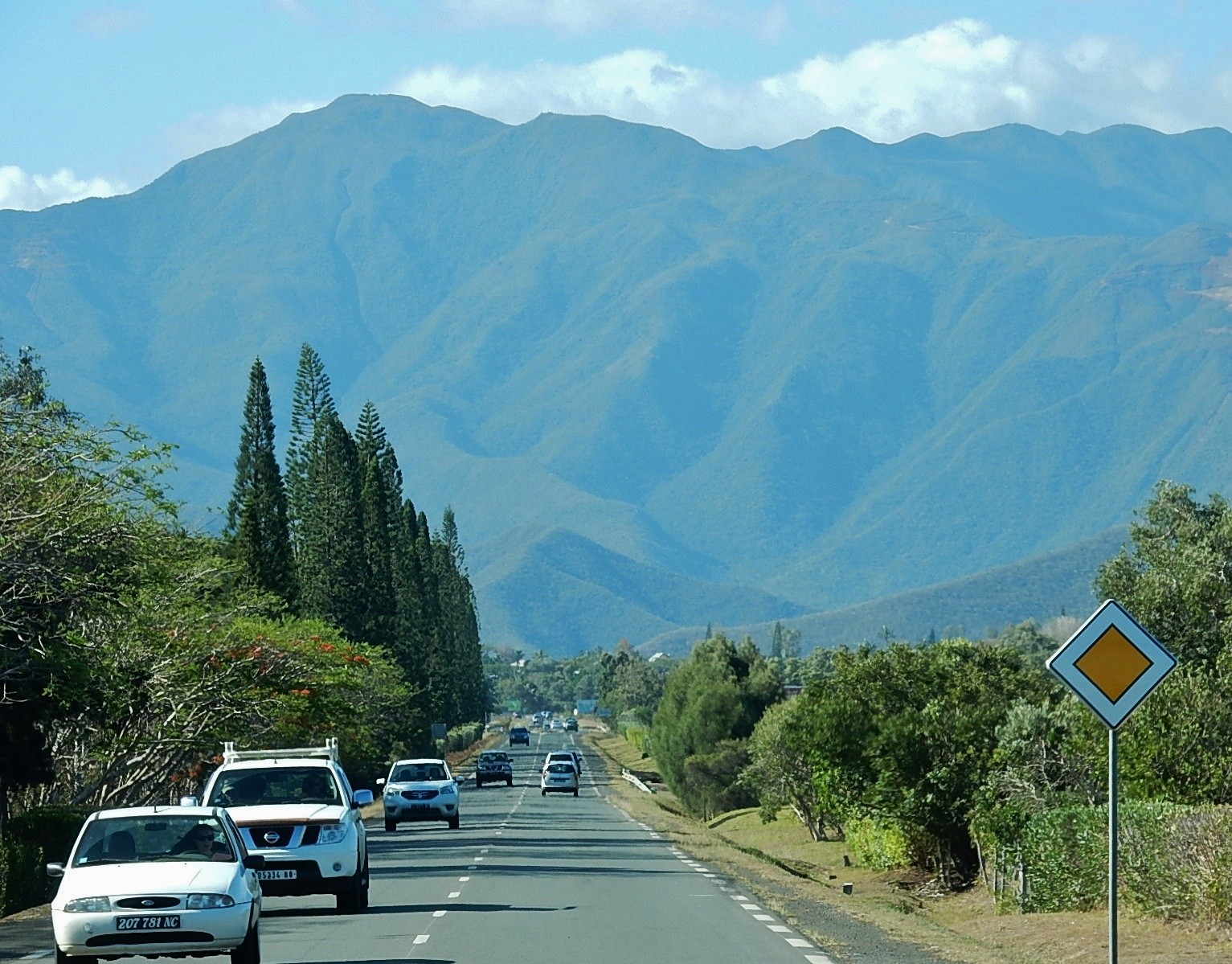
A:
[542, 751, 583, 779]
[376, 758, 465, 830]
[474, 750, 514, 787]
[180, 741, 373, 913]
[508, 727, 531, 747]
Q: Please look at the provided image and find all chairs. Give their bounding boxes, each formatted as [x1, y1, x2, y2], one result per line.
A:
[228, 773, 267, 805]
[400, 770, 415, 782]
[98, 831, 137, 861]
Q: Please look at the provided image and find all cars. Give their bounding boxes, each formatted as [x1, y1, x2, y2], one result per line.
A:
[47, 800, 264, 964]
[539, 760, 581, 796]
[512, 710, 578, 732]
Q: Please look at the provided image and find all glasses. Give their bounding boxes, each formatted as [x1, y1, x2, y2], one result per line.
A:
[194, 835, 215, 841]
[310, 782, 322, 787]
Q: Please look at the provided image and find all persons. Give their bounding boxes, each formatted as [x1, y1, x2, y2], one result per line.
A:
[431, 769, 439, 779]
[190, 823, 217, 859]
